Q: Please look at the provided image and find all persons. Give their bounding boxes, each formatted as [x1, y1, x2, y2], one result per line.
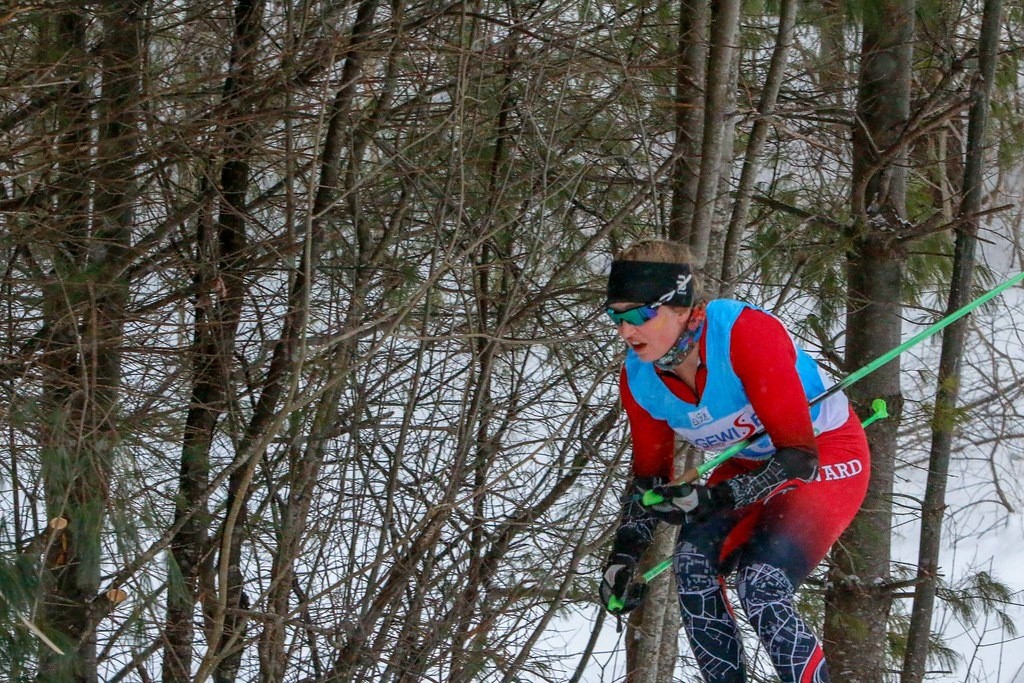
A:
[599, 241, 870, 683]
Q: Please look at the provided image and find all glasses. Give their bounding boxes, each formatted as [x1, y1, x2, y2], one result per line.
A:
[605, 272, 693, 327]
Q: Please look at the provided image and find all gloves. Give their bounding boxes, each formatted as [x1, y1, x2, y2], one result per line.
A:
[631, 480, 734, 528]
[598, 539, 650, 614]
